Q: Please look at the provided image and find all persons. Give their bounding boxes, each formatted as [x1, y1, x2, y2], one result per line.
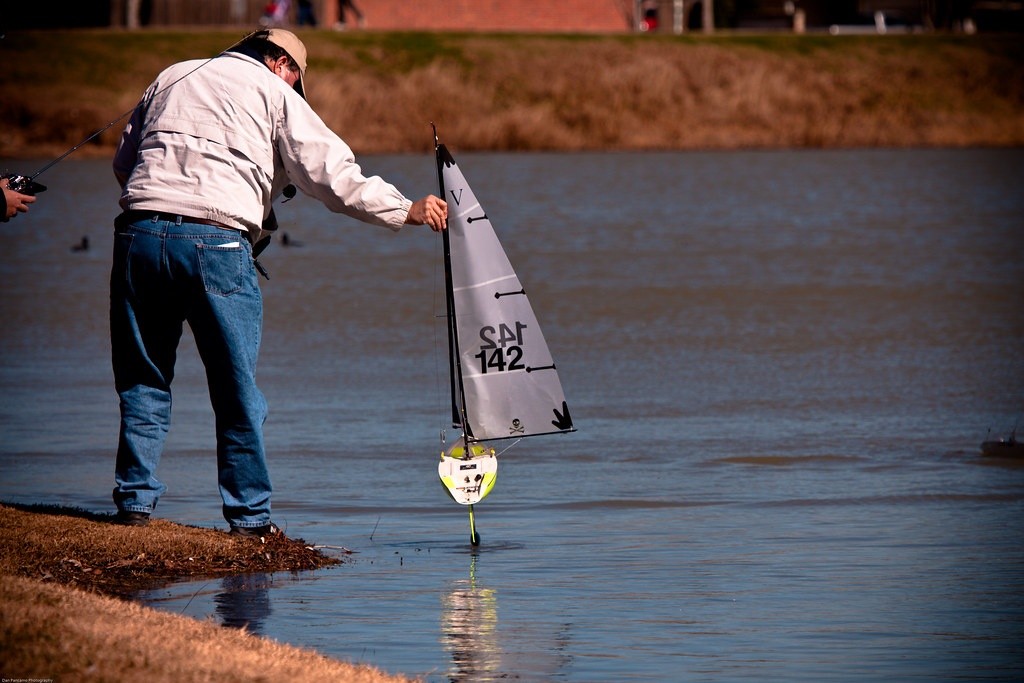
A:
[257, 0, 366, 30]
[0, 177, 37, 223]
[110, 29, 448, 536]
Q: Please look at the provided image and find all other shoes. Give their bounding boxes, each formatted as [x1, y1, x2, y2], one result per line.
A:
[333, 23, 347, 32]
[230, 523, 284, 537]
[117, 510, 147, 526]
[357, 15, 368, 29]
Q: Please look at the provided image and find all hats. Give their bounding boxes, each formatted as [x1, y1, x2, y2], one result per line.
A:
[257, 29, 307, 102]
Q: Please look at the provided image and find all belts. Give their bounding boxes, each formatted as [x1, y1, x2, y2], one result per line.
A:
[124, 208, 231, 228]
